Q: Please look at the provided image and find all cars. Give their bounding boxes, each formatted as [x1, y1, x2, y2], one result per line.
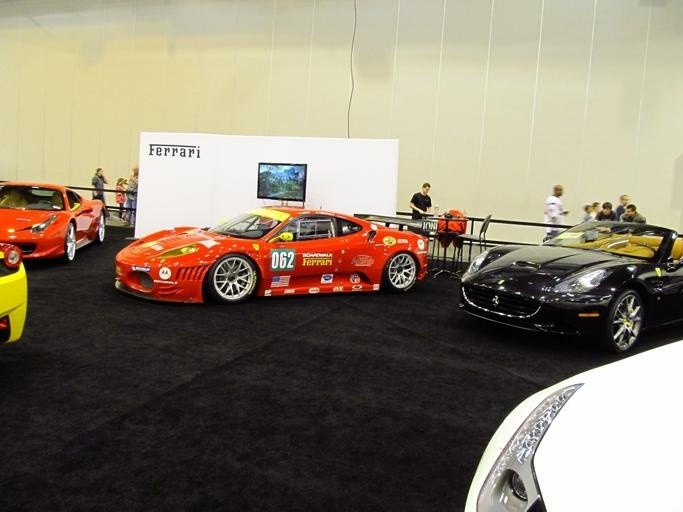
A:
[462, 338, 682, 512]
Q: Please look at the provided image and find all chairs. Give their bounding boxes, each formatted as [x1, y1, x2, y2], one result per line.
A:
[454, 213, 492, 276]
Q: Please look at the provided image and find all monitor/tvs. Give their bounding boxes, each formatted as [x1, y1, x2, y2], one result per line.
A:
[257, 162, 307, 202]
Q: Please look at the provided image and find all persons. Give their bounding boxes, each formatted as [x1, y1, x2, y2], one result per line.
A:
[116, 179, 125, 219]
[92, 168, 109, 217]
[410, 183, 431, 219]
[583, 196, 645, 242]
[544, 186, 567, 239]
[122, 168, 139, 225]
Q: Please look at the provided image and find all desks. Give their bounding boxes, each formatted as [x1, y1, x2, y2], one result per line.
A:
[427, 217, 469, 281]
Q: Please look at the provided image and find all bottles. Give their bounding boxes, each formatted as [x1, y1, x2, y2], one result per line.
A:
[433, 205, 439, 218]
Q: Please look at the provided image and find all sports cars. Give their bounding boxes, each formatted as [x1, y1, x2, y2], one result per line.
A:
[0, 241, 29, 345]
[0, 181, 109, 261]
[456, 220, 683, 353]
[111, 204, 439, 304]
